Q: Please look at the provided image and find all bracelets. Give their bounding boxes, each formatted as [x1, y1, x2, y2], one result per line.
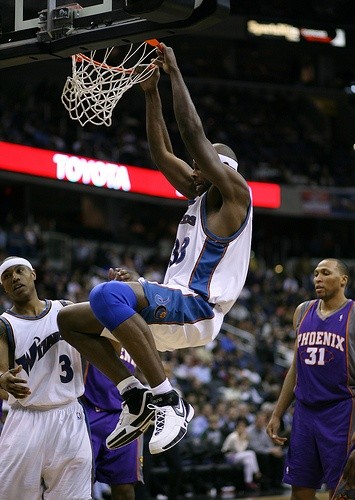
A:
[0, 373, 6, 391]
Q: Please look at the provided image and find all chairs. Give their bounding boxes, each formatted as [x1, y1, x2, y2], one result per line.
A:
[144, 442, 240, 500]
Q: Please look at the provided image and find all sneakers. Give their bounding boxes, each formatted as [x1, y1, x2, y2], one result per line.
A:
[148, 389, 195, 456]
[105, 390, 154, 450]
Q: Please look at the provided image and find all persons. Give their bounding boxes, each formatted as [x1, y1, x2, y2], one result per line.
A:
[138, 217, 354, 499]
[58, 43, 254, 453]
[0, 255, 131, 499]
[1, 183, 180, 302]
[59, 265, 145, 499]
[1, 34, 355, 185]
